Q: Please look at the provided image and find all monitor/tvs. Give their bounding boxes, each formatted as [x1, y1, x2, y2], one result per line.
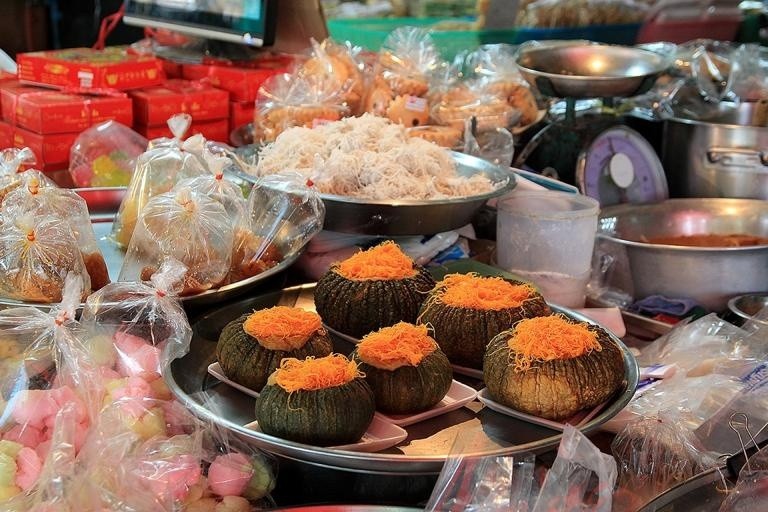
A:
[122, 0, 278, 63]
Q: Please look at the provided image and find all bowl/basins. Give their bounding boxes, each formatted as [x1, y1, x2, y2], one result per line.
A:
[727, 293, 768, 333]
[595, 197, 768, 312]
[222, 139, 516, 236]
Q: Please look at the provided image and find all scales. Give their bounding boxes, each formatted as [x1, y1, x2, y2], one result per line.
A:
[515, 45, 669, 214]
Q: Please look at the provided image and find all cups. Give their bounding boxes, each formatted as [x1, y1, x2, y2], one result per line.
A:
[496, 190, 599, 309]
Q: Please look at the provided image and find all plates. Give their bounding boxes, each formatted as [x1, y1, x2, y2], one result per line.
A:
[476, 386, 614, 432]
[205, 361, 267, 400]
[373, 377, 478, 423]
[237, 412, 410, 455]
[0, 184, 324, 316]
[163, 283, 639, 468]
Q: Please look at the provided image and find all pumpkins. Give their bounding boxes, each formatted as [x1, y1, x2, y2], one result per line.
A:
[417, 274, 547, 362]
[215, 310, 333, 391]
[313, 264, 433, 336]
[254, 372, 376, 446]
[348, 333, 451, 415]
[483, 317, 626, 420]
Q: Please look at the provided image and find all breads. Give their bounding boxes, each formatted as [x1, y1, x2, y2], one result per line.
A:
[260, 49, 392, 138]
[387, 78, 536, 149]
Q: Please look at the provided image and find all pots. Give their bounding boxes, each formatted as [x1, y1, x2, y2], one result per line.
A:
[661, 115, 767, 202]
